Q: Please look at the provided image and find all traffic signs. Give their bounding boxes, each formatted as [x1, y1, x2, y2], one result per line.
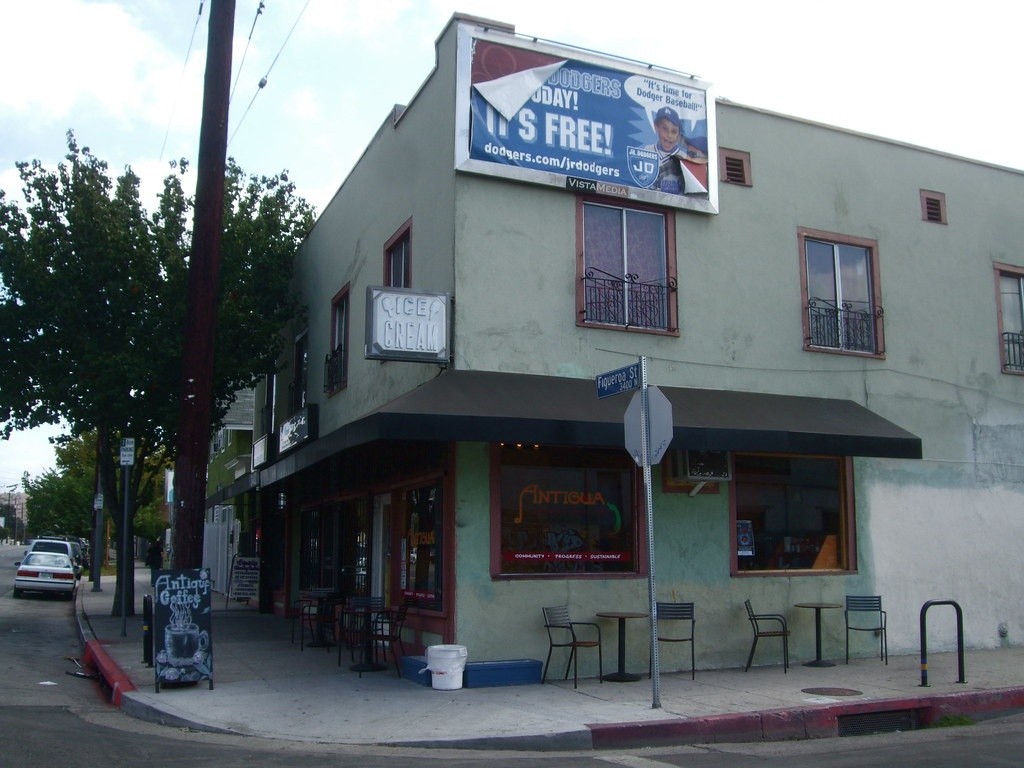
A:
[119, 437, 135, 466]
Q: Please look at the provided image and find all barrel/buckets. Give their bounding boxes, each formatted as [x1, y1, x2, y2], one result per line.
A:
[426, 644, 468, 689]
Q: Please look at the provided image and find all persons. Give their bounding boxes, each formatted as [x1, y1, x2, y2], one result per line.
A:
[644, 106, 708, 196]
[146, 541, 163, 587]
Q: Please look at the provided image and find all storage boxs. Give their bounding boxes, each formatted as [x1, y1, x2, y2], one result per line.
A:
[401, 656, 432, 687]
[464, 659, 543, 688]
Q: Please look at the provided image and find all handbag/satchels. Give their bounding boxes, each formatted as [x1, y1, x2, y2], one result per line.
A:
[145, 557, 153, 565]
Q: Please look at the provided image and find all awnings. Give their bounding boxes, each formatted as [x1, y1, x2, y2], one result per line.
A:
[258, 371, 923, 488]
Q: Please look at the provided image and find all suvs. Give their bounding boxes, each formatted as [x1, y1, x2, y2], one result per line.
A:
[24, 538, 80, 570]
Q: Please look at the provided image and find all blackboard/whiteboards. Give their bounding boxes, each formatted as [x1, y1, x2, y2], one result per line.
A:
[152, 568, 213, 694]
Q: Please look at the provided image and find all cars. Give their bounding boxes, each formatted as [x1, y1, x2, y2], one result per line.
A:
[13, 551, 80, 599]
[26, 534, 90, 570]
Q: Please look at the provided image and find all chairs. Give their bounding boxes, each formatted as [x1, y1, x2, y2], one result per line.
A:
[648, 603, 696, 680]
[744, 600, 790, 674]
[542, 606, 604, 689]
[844, 595, 888, 667]
[298, 588, 410, 679]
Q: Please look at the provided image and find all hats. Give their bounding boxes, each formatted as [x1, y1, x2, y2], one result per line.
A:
[655, 107, 681, 127]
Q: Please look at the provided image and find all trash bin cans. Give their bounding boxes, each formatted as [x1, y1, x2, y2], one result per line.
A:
[428, 644, 467, 690]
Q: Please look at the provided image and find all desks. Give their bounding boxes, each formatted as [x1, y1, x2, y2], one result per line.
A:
[794, 603, 842, 668]
[304, 593, 342, 647]
[344, 606, 395, 672]
[596, 613, 647, 683]
